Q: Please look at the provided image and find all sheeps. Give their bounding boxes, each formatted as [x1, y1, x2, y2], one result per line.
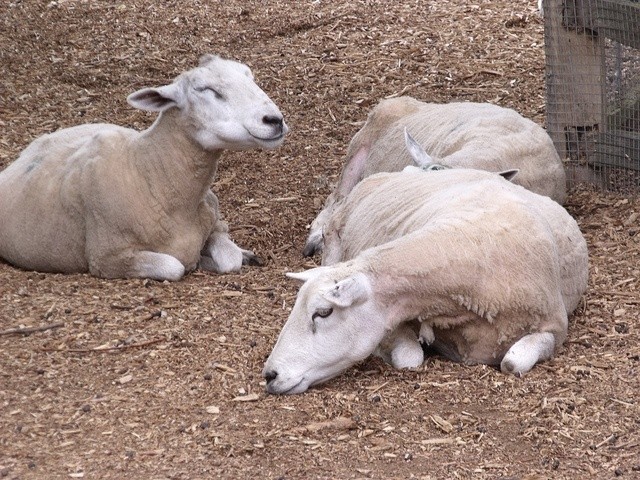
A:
[261, 164, 590, 395]
[1, 52, 289, 281]
[302, 95, 567, 259]
